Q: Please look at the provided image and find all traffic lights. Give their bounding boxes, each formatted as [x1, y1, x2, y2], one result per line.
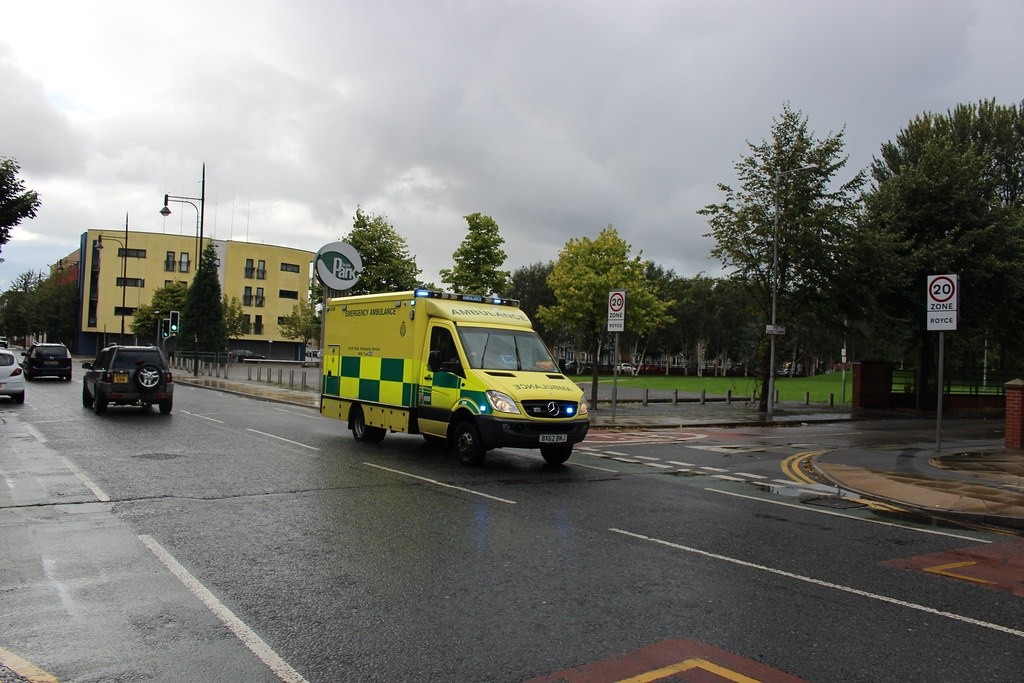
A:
[161, 319, 171, 339]
[168, 310, 181, 333]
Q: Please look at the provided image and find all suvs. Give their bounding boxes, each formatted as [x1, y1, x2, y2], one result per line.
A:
[81, 342, 175, 416]
[21, 342, 72, 381]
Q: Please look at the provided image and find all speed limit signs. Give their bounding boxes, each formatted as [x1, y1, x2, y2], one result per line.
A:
[927, 274, 959, 331]
[608, 289, 625, 332]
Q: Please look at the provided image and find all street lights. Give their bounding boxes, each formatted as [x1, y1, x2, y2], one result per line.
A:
[95, 235, 129, 347]
[160, 193, 205, 391]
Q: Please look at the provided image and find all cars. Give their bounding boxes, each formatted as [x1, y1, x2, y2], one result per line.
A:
[0, 349, 26, 404]
[0, 336, 8, 349]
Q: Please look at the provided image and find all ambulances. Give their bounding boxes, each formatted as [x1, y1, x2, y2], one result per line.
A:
[319, 289, 590, 467]
